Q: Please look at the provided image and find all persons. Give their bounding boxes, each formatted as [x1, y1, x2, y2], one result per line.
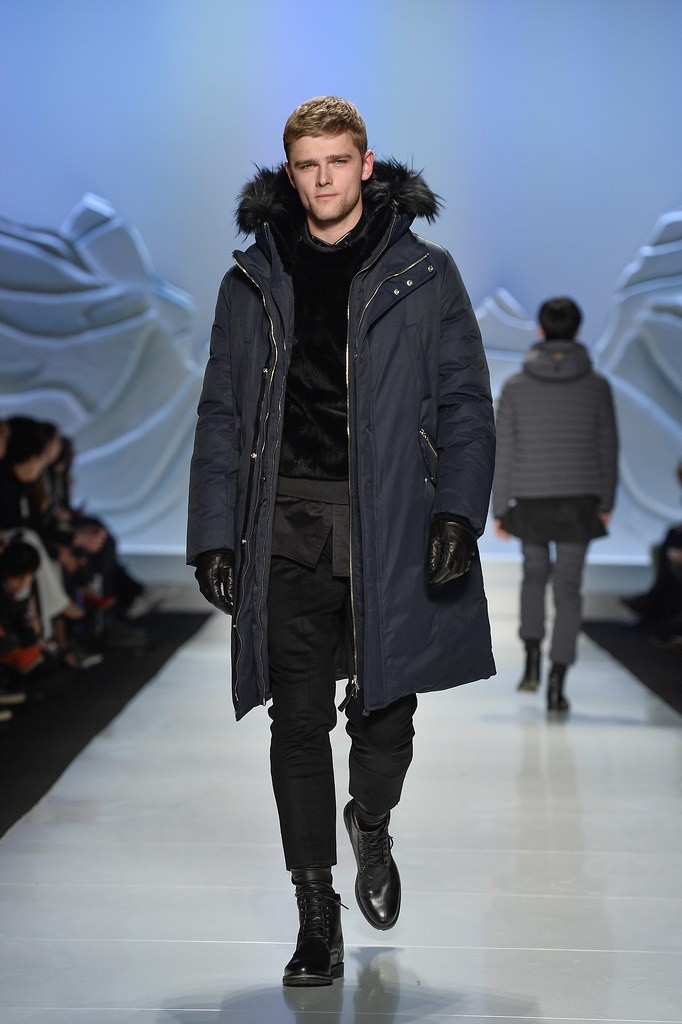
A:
[492, 297, 619, 714]
[186, 95, 499, 987]
[1, 413, 144, 734]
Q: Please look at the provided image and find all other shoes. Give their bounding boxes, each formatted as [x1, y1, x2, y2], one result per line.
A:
[518, 650, 541, 693]
[547, 672, 568, 711]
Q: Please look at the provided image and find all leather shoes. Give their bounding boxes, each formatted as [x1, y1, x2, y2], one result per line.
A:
[282, 893, 344, 987]
[343, 799, 402, 931]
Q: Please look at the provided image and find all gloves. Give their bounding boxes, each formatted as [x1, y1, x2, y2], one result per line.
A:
[193, 548, 237, 616]
[426, 514, 478, 586]
